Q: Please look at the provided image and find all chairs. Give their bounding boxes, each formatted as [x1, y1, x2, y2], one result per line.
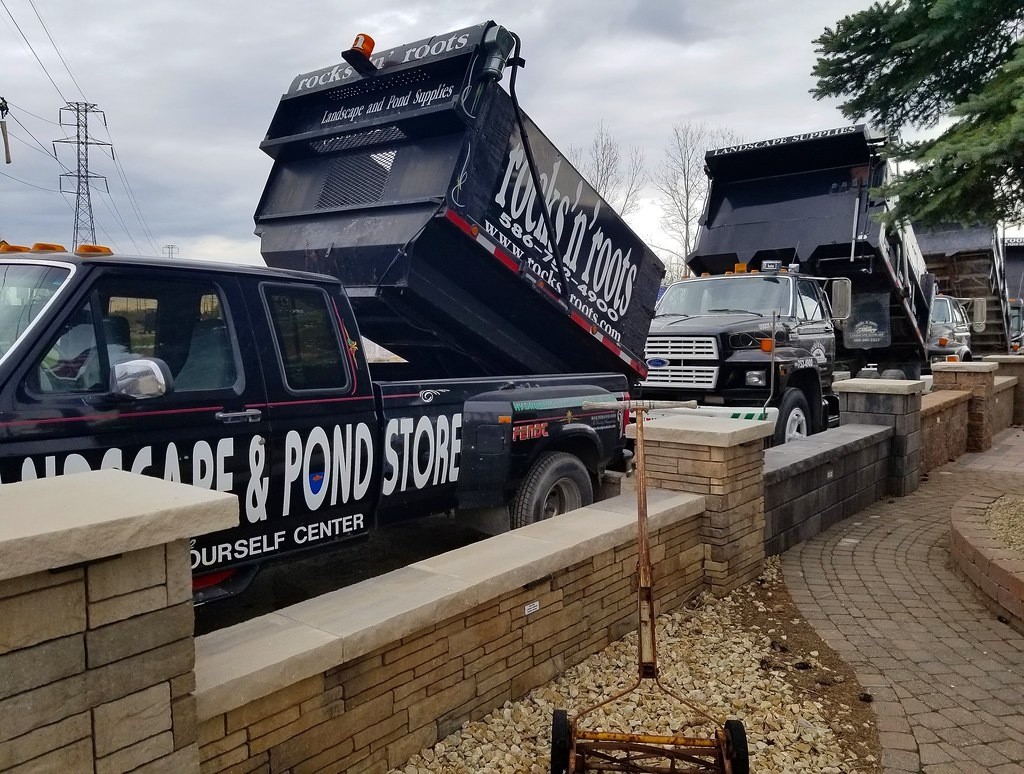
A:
[174, 317, 234, 393]
[103, 315, 133, 373]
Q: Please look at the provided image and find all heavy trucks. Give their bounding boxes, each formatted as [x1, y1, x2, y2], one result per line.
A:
[905, 217, 1024, 366]
[634, 124, 939, 449]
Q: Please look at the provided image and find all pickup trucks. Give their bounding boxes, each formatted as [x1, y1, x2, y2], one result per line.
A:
[0, 18, 641, 620]
